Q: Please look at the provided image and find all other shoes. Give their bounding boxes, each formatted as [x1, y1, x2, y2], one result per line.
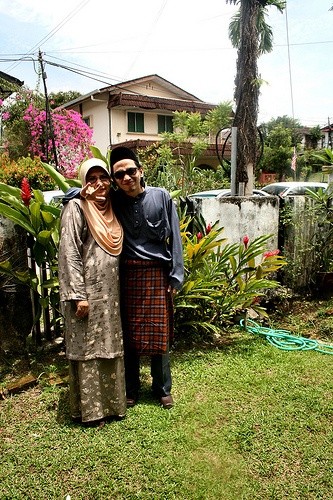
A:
[89, 420, 106, 427]
[116, 415, 125, 420]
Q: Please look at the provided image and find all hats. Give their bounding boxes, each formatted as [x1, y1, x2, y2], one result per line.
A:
[110, 146, 139, 167]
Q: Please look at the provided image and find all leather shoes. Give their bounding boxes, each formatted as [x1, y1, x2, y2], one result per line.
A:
[160, 396, 173, 409]
[127, 399, 136, 406]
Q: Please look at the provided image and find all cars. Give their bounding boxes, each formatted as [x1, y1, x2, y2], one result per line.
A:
[186, 188, 273, 197]
[258, 181, 330, 197]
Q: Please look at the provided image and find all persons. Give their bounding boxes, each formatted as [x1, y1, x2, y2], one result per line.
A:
[53, 146, 185, 408]
[56, 158, 129, 427]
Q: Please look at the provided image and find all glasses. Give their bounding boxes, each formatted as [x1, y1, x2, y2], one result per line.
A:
[111, 167, 138, 179]
[89, 174, 109, 183]
